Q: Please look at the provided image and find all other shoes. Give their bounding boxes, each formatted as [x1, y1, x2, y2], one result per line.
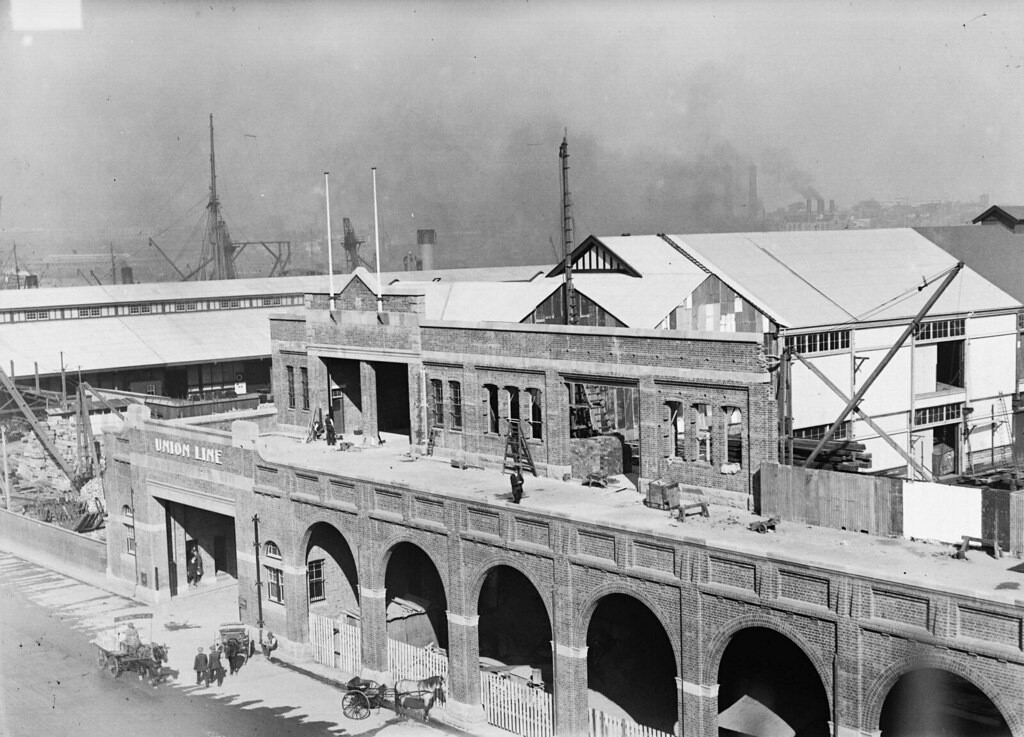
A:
[328, 443, 330, 445]
[332, 443, 335, 445]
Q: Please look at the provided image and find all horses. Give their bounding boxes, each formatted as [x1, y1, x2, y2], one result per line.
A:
[135, 643, 169, 688]
[221, 638, 241, 675]
[394, 673, 448, 724]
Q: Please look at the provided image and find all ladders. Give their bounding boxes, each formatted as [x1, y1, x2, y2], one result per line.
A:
[502, 419, 538, 476]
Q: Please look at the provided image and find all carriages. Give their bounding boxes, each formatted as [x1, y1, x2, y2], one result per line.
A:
[91, 630, 170, 690]
[340, 675, 445, 724]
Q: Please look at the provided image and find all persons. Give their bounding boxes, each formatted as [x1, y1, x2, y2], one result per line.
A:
[313, 421, 323, 441]
[193, 647, 209, 687]
[193, 550, 202, 583]
[324, 414, 337, 446]
[208, 645, 222, 686]
[225, 640, 238, 674]
[126, 623, 142, 644]
[261, 631, 278, 658]
[510, 466, 524, 504]
[187, 555, 199, 587]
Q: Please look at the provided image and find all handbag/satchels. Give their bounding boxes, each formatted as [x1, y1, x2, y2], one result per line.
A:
[196, 569, 204, 576]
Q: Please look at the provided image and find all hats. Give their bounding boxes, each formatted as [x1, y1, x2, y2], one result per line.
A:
[128, 623, 133, 627]
[512, 465, 520, 470]
[326, 414, 329, 416]
[210, 645, 216, 649]
[198, 647, 202, 651]
[314, 421, 319, 423]
[267, 632, 272, 638]
